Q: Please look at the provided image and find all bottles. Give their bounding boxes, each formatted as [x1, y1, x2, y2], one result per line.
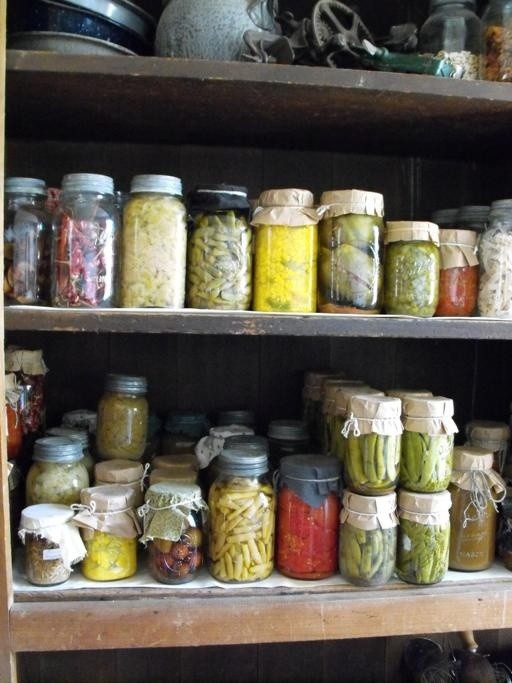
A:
[414, 3, 510, 81]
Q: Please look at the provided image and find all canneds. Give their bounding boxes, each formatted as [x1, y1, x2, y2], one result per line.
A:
[50, 173, 120, 308]
[435, 229, 482, 316]
[431, 208, 458, 229]
[5, 350, 511, 585]
[115, 191, 129, 223]
[4, 177, 48, 304]
[457, 206, 490, 233]
[45, 187, 62, 213]
[316, 189, 385, 314]
[384, 221, 440, 318]
[119, 175, 188, 308]
[249, 188, 324, 313]
[187, 184, 252, 310]
[477, 199, 512, 317]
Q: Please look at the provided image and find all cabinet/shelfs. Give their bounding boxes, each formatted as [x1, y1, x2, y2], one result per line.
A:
[0, 0, 512, 683]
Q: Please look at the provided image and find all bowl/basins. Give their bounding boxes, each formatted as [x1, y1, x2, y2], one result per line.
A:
[12, 0, 157, 59]
